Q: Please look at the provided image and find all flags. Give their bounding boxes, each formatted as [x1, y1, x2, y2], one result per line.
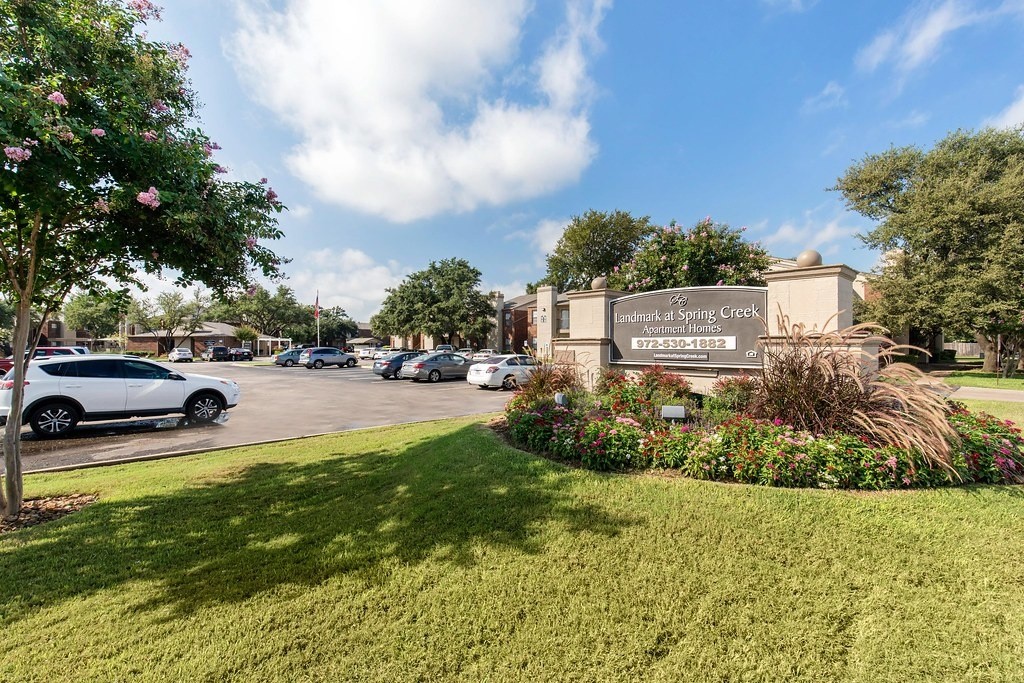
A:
[315, 295, 319, 320]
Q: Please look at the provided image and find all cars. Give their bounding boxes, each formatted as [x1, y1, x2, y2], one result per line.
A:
[200, 346, 229, 361]
[298, 347, 357, 370]
[271, 348, 305, 367]
[168, 347, 193, 363]
[223, 347, 254, 362]
[293, 344, 316, 349]
[0, 342, 90, 382]
[0, 352, 240, 438]
[360, 342, 562, 393]
